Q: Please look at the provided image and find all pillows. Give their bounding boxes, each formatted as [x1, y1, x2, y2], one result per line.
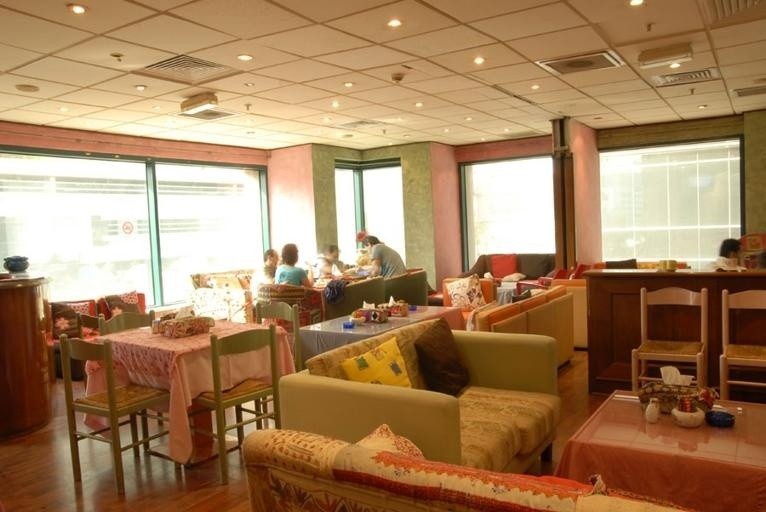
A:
[356, 423, 414, 457]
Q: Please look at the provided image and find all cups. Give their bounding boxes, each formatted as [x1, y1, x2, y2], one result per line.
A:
[658, 260, 677, 272]
[538, 277, 553, 287]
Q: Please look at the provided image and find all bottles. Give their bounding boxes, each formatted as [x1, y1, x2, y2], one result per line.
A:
[4, 256, 30, 273]
[151, 320, 160, 334]
[644, 397, 662, 423]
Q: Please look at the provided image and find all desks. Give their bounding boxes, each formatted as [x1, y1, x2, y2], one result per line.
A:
[583, 266, 766, 394]
[554, 387, 766, 511]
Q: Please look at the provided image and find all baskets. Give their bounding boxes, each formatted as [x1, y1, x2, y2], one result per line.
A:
[637, 380, 717, 416]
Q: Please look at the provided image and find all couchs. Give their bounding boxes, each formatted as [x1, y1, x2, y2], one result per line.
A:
[241, 428, 686, 511]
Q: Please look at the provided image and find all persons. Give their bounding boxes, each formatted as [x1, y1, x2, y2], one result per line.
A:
[275, 244, 313, 288]
[313, 245, 341, 278]
[250, 250, 279, 306]
[710, 239, 743, 270]
[365, 236, 406, 277]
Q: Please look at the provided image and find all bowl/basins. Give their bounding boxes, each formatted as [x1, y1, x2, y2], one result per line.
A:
[671, 406, 707, 428]
[705, 411, 736, 427]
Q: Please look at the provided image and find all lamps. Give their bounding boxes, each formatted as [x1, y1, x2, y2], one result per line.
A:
[177, 92, 222, 116]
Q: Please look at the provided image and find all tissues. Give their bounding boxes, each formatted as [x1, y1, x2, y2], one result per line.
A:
[636, 367, 713, 419]
[162, 304, 214, 338]
[357, 301, 388, 323]
[484, 272, 501, 286]
[378, 296, 410, 317]
[330, 264, 343, 280]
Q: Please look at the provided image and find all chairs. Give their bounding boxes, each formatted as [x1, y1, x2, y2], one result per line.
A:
[41, 251, 587, 494]
[631, 287, 708, 399]
[718, 288, 766, 404]
